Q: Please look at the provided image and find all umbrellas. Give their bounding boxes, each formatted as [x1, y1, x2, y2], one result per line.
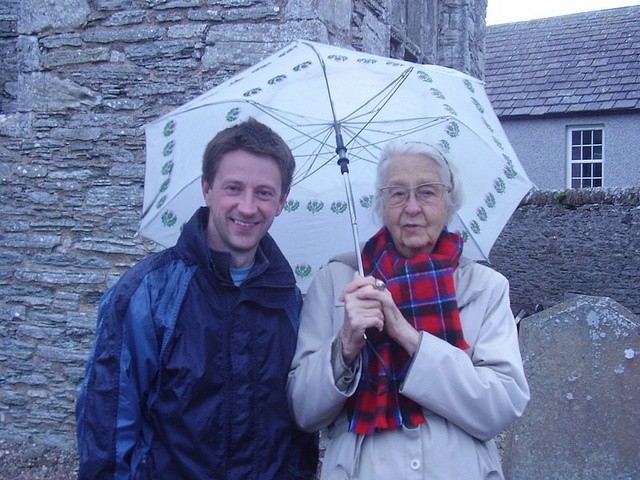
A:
[133, 39, 539, 295]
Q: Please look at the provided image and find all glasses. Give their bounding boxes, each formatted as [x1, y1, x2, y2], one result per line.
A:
[378, 183, 450, 207]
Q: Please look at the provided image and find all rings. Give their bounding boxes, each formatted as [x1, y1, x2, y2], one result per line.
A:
[375, 278, 386, 291]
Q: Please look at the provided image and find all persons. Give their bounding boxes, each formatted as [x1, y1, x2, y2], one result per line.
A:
[76, 117, 318, 479]
[285, 139, 530, 480]
